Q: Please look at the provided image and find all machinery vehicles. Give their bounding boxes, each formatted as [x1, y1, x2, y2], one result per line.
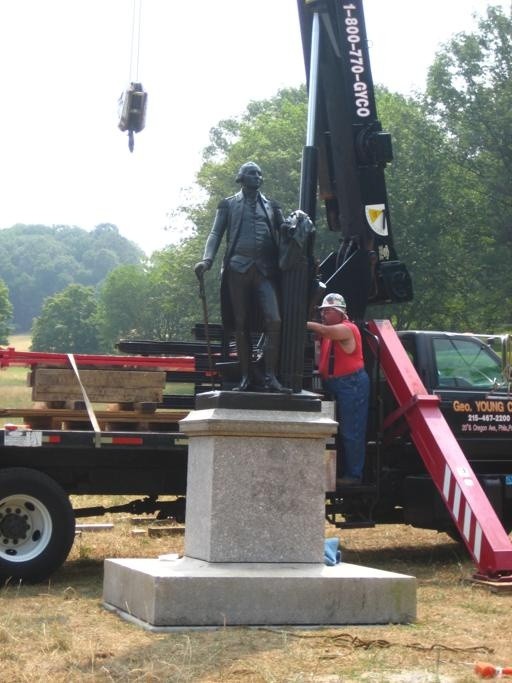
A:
[0, 17, 511, 587]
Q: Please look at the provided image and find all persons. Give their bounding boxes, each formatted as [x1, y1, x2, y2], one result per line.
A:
[192, 159, 309, 394]
[306, 291, 373, 486]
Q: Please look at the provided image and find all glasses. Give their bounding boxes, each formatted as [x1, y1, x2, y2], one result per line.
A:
[321, 309, 341, 314]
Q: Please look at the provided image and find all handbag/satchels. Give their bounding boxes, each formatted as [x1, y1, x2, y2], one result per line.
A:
[324, 540, 342, 566]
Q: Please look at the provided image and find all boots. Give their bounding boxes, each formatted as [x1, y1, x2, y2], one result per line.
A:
[231, 349, 252, 390]
[264, 348, 293, 393]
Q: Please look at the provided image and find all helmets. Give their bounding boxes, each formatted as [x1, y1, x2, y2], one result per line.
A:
[318, 293, 349, 308]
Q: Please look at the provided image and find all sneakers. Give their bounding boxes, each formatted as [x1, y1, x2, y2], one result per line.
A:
[337, 476, 365, 486]
